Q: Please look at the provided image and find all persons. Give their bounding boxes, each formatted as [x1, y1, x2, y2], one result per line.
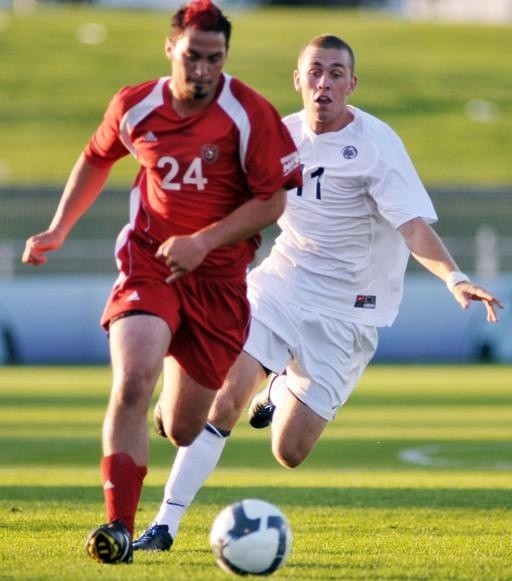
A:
[20, 0, 303, 564]
[132, 34, 505, 551]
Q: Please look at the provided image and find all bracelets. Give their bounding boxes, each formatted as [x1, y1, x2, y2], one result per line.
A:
[447, 271, 470, 292]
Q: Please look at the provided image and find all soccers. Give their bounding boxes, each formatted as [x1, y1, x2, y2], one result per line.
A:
[210, 499, 291, 576]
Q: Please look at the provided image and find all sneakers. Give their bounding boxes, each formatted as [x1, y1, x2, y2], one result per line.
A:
[132, 524, 173, 551]
[248, 367, 286, 429]
[86, 519, 132, 563]
